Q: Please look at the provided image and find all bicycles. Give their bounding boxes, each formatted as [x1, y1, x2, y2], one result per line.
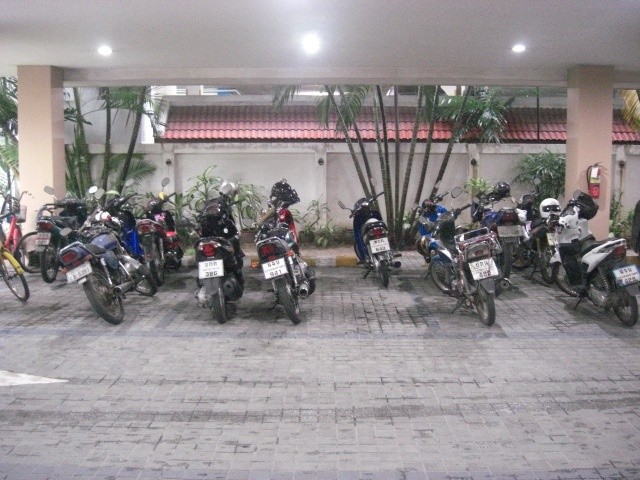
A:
[16, 229, 54, 273]
[1, 248, 29, 302]
[1, 192, 28, 244]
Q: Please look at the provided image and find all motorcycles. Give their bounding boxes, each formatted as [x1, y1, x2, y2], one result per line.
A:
[131, 191, 188, 286]
[59, 191, 159, 325]
[188, 180, 247, 326]
[506, 176, 562, 284]
[466, 188, 521, 285]
[336, 176, 403, 288]
[544, 189, 640, 328]
[249, 178, 316, 325]
[35, 186, 87, 286]
[410, 190, 505, 330]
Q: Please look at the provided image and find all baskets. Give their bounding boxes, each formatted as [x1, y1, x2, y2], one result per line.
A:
[6, 205, 26, 222]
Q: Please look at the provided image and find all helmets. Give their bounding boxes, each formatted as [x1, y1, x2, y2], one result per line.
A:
[269, 181, 300, 209]
[219, 180, 238, 199]
[539, 198, 562, 219]
[494, 182, 510, 195]
[567, 190, 599, 220]
